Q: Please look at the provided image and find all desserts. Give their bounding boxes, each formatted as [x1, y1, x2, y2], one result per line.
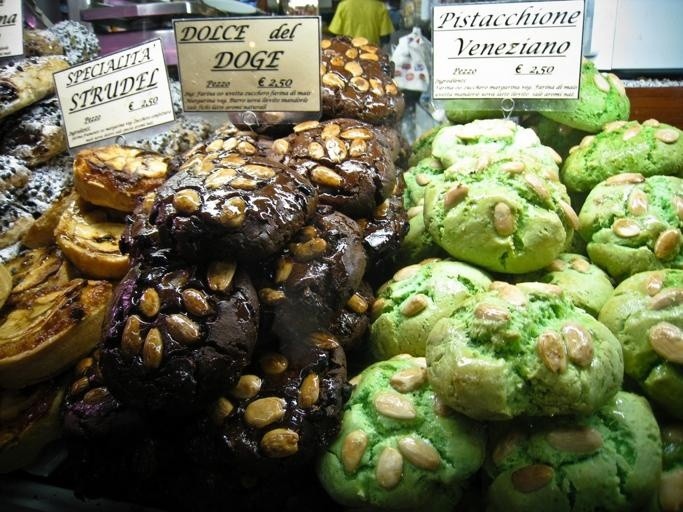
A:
[0, 20, 683, 512]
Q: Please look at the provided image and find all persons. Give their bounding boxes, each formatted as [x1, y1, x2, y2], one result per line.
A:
[388, 0, 405, 30]
[327, 0, 395, 48]
